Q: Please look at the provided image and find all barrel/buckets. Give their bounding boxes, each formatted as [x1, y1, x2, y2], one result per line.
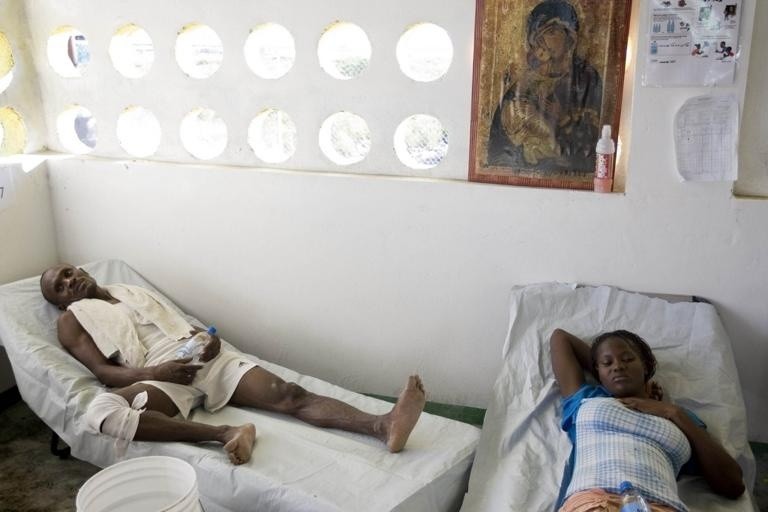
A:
[74, 456, 205, 512]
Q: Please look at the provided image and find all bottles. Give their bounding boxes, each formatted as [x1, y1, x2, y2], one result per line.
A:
[173, 326, 217, 366]
[591, 122, 617, 193]
[619, 480, 648, 512]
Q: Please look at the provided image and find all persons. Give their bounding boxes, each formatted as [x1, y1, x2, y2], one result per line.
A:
[715, 41, 734, 58]
[40, 263, 428, 466]
[690, 43, 704, 56]
[550, 328, 745, 512]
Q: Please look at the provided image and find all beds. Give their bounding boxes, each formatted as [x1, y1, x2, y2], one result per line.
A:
[0, 258, 481, 512]
[460, 282, 757, 512]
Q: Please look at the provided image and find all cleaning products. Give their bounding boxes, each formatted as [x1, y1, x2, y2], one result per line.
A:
[594, 126, 615, 192]
